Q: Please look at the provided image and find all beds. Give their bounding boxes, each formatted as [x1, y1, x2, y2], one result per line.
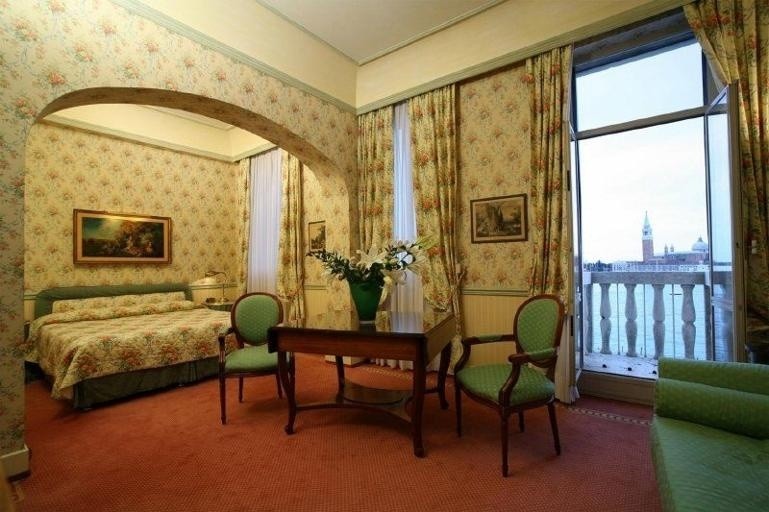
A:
[20, 283, 251, 414]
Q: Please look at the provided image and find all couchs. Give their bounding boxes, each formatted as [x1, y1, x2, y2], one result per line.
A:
[648, 356, 769, 511]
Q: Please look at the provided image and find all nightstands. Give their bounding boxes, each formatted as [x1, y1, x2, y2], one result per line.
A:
[201, 302, 235, 311]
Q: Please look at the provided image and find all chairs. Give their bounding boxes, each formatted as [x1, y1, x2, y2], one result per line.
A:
[217, 291, 295, 423]
[454, 295, 565, 478]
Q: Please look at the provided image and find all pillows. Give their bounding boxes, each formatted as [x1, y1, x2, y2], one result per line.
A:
[654, 376, 769, 439]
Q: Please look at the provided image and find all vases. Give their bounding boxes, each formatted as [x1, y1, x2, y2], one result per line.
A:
[349, 282, 384, 322]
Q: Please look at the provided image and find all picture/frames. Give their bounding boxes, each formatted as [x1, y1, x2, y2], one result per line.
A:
[73, 208, 172, 265]
[470, 193, 529, 243]
[308, 220, 325, 253]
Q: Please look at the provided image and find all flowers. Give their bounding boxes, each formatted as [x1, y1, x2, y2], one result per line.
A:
[306, 234, 439, 305]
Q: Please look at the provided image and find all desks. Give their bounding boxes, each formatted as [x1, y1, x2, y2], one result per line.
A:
[267, 310, 456, 458]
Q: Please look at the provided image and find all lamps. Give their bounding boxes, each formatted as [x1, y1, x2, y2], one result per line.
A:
[205, 271, 230, 302]
